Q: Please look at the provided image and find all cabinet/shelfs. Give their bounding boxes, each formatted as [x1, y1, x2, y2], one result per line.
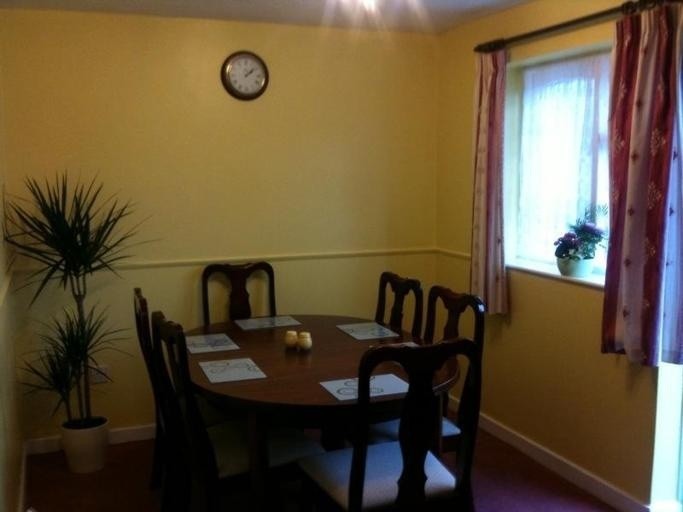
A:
[298, 332, 312, 351]
[284, 330, 298, 348]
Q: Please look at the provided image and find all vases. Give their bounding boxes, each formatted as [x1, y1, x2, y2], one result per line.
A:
[553, 204, 610, 265]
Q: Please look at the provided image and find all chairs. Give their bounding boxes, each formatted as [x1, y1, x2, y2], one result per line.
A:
[203, 262, 277, 424]
[294, 337, 481, 511]
[151, 311, 271, 511]
[134, 287, 182, 495]
[321, 285, 485, 453]
[295, 271, 424, 441]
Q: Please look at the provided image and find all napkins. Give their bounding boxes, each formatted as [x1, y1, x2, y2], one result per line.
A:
[184, 332, 240, 355]
[198, 357, 268, 384]
[319, 373, 410, 401]
[234, 315, 302, 332]
[234, 316, 302, 331]
[370, 341, 421, 366]
[336, 321, 400, 340]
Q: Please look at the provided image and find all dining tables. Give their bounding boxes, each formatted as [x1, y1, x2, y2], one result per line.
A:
[1, 167, 162, 475]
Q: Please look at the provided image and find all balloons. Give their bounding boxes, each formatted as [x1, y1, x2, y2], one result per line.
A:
[220, 50, 269, 100]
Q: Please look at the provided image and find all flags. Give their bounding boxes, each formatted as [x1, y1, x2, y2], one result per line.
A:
[558, 255, 596, 278]
